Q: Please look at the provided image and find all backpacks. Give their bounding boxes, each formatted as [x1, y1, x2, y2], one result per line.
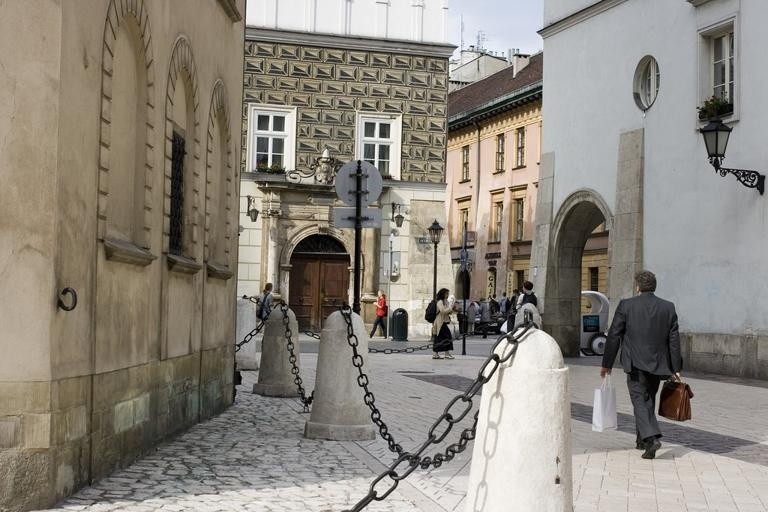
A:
[505, 298, 511, 312]
[425, 299, 444, 323]
[522, 292, 537, 307]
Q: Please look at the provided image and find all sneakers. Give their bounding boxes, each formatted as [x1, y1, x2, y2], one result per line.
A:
[467, 331, 488, 340]
[445, 355, 455, 359]
[432, 355, 444, 359]
[369, 334, 388, 339]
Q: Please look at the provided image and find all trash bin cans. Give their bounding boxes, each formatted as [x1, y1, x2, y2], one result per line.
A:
[456, 314, 467, 334]
[391, 308, 408, 341]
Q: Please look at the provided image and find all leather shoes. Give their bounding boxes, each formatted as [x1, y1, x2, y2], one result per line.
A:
[642, 438, 662, 459]
[636, 439, 645, 450]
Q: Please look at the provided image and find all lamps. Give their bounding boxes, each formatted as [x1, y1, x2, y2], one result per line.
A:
[695, 116, 765, 198]
[389, 200, 405, 229]
[246, 194, 260, 223]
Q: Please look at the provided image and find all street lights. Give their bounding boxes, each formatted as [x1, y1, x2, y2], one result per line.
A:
[426, 218, 445, 299]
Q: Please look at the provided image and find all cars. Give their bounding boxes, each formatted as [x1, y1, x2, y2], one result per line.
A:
[453, 299, 506, 336]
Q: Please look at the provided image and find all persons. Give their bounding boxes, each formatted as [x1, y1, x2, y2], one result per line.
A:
[600, 270, 683, 458]
[455, 281, 537, 336]
[433, 288, 456, 358]
[259, 283, 274, 350]
[369, 289, 388, 338]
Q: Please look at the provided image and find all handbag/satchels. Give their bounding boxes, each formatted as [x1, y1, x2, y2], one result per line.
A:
[384, 306, 388, 318]
[591, 373, 619, 432]
[658, 374, 694, 422]
[256, 306, 265, 318]
